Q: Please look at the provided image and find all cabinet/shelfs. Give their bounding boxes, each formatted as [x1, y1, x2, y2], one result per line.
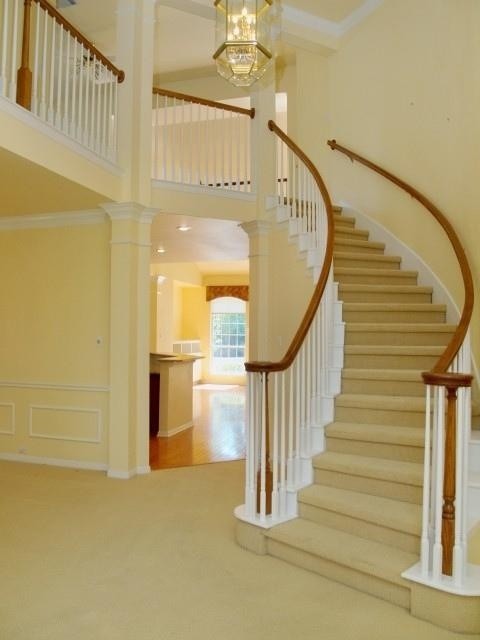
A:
[173, 340, 202, 386]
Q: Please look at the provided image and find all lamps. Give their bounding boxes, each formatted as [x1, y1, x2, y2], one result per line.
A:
[212, 1, 273, 89]
